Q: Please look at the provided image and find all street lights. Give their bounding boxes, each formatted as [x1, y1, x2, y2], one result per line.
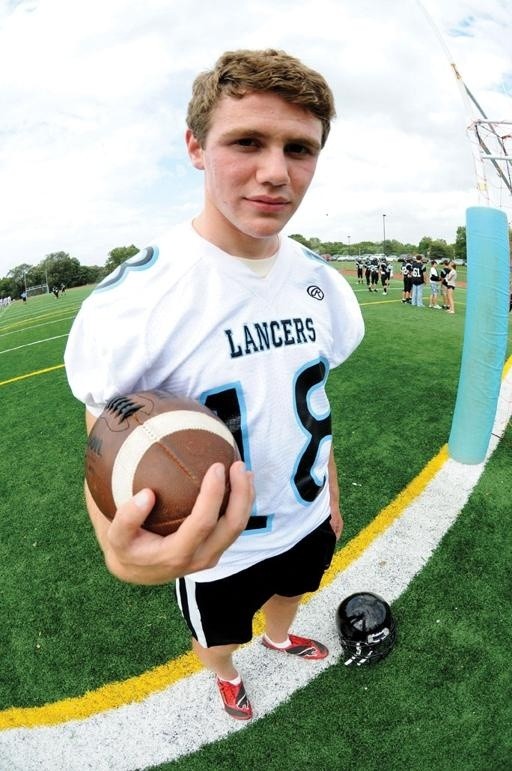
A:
[347, 235, 351, 256]
[383, 214, 386, 254]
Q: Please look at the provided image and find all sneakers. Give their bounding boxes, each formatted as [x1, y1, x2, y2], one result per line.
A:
[402, 297, 425, 307]
[216, 676, 252, 720]
[369, 286, 388, 295]
[429, 303, 455, 314]
[261, 633, 329, 659]
[358, 279, 363, 284]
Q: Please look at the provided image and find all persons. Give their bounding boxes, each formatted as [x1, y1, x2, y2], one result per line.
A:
[60, 283, 65, 295]
[53, 285, 56, 295]
[55, 287, 60, 298]
[355, 255, 457, 315]
[62, 46, 369, 722]
[21, 292, 28, 304]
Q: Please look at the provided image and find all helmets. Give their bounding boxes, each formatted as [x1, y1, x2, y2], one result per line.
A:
[337, 591, 396, 665]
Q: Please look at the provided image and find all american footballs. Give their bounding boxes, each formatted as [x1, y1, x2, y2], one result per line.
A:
[86, 391, 241, 536]
